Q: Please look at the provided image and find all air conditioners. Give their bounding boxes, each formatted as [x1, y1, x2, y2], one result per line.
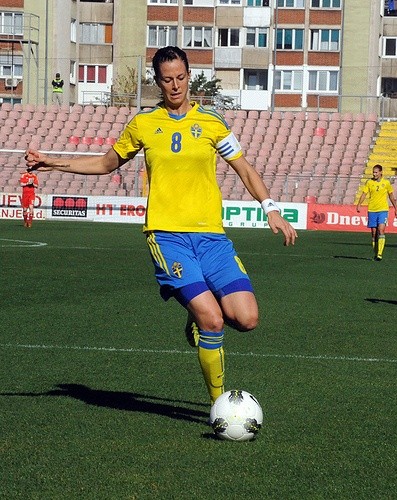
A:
[4, 78, 17, 87]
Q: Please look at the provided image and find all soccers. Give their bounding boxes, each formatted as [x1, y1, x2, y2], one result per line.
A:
[210, 389, 265, 443]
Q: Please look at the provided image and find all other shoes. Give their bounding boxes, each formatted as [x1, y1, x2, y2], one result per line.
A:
[24, 222, 32, 228]
[374, 253, 383, 261]
[185, 312, 201, 347]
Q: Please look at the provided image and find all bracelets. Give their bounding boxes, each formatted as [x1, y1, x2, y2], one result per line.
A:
[261, 198, 279, 213]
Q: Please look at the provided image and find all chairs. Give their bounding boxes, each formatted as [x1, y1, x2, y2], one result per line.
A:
[0, 102, 397, 208]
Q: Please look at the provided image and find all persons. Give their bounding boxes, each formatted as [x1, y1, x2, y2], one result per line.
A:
[25, 46, 298, 415]
[51, 73, 64, 105]
[356, 164, 397, 261]
